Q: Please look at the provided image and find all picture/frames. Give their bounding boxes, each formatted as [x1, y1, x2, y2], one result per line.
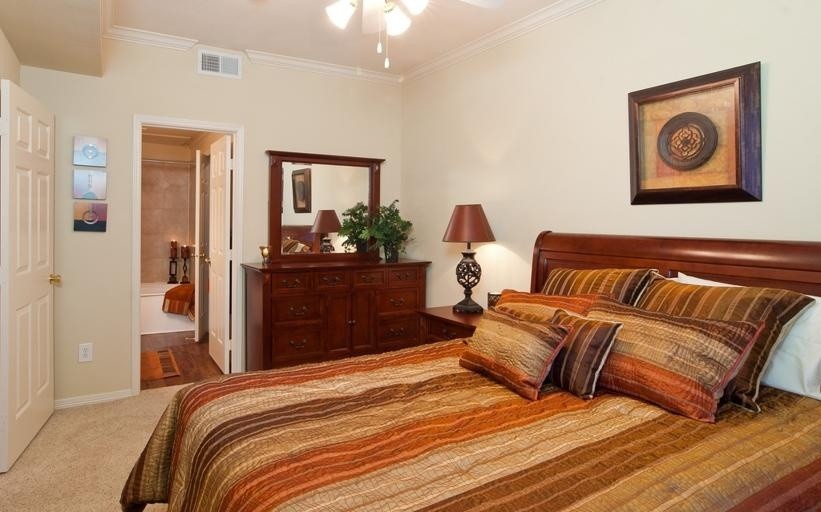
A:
[627, 61, 762, 205]
[291, 168, 311, 213]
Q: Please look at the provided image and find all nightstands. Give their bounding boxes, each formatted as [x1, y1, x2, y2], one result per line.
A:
[412, 304, 485, 345]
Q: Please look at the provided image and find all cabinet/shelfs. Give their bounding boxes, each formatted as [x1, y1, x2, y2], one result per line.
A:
[240, 257, 431, 371]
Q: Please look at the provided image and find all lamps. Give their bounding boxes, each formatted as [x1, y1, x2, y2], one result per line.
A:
[442, 203, 496, 314]
[308, 209, 342, 253]
[166, 239, 179, 284]
[179, 245, 190, 284]
[324, 0, 429, 68]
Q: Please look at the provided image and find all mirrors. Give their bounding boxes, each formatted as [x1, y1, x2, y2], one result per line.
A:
[264, 149, 386, 263]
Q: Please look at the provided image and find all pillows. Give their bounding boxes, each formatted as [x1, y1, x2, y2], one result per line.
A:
[494, 288, 615, 325]
[580, 295, 766, 427]
[539, 266, 660, 308]
[542, 307, 625, 401]
[634, 270, 815, 414]
[458, 306, 574, 402]
[665, 271, 819, 401]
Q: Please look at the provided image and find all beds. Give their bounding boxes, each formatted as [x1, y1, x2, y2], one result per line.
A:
[160, 283, 196, 321]
[281, 224, 320, 254]
[116, 228, 820, 512]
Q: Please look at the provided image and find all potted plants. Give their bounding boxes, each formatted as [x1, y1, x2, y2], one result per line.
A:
[335, 201, 367, 254]
[357, 199, 415, 263]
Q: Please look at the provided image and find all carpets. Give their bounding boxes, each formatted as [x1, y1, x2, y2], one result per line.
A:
[140, 348, 180, 383]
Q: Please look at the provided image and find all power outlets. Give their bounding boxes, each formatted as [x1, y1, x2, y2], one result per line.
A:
[79, 342, 93, 364]
